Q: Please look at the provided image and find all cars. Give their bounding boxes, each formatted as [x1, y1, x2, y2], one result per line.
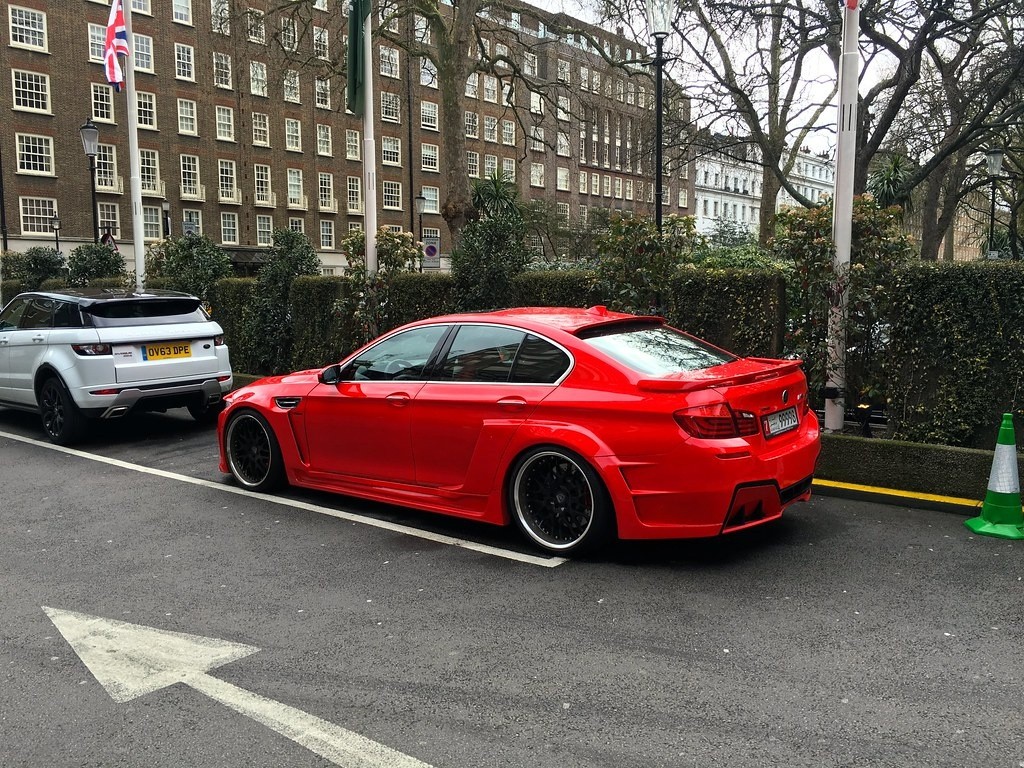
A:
[215, 305, 822, 563]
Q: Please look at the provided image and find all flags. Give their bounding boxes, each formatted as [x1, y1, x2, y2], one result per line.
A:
[346, 1, 371, 117]
[104, 1, 130, 95]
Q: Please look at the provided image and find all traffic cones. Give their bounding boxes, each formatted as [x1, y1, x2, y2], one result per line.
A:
[967, 412, 1024, 539]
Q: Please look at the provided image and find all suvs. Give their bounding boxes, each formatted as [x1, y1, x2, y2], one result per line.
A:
[0, 284, 236, 444]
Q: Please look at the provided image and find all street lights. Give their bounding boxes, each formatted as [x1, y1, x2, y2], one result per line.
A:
[47, 211, 62, 253]
[984, 141, 1005, 254]
[161, 197, 171, 239]
[80, 115, 106, 246]
[639, 0, 677, 249]
[415, 191, 428, 275]
